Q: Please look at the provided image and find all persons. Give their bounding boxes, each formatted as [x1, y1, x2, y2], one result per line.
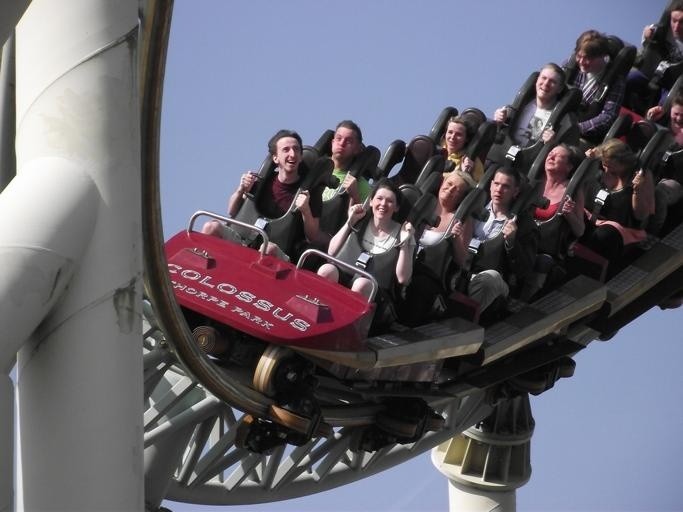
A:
[202, 120, 414, 337]
[413, 3, 682, 325]
